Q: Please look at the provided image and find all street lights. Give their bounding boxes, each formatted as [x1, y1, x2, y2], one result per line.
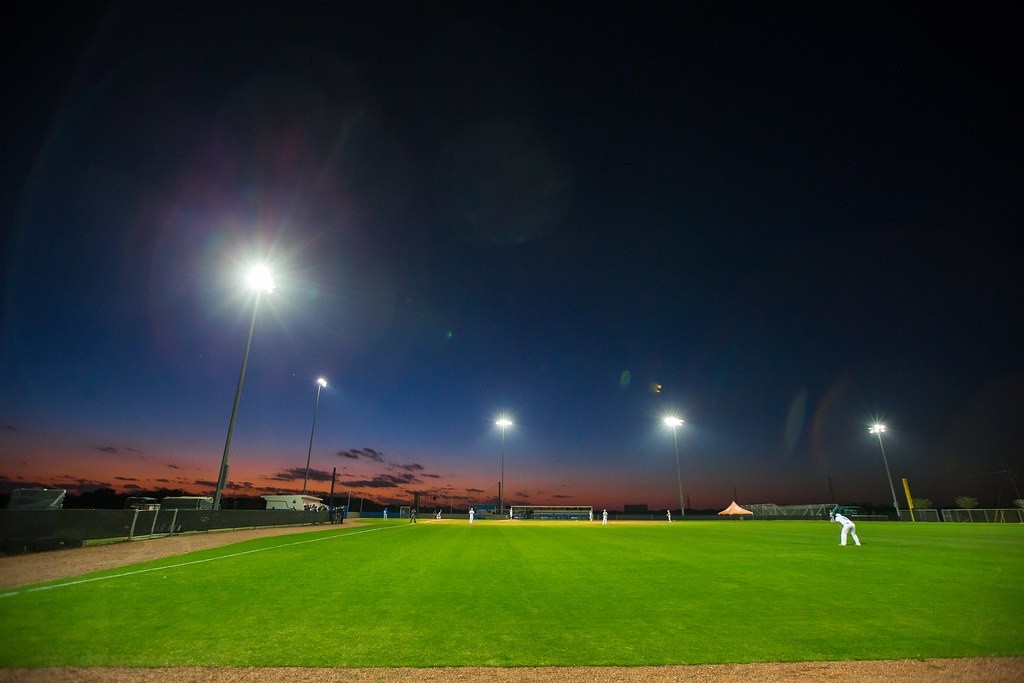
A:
[496, 420, 512, 515]
[303, 378, 327, 495]
[212, 263, 275, 509]
[868, 424, 901, 516]
[665, 417, 685, 516]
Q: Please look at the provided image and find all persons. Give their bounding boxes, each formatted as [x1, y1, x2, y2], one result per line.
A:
[602, 509, 608, 525]
[303, 504, 346, 524]
[383, 507, 388, 521]
[589, 511, 593, 521]
[433, 510, 441, 519]
[410, 508, 417, 523]
[666, 509, 671, 522]
[469, 508, 475, 523]
[829, 512, 861, 546]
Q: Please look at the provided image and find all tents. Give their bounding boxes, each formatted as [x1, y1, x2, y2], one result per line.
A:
[718, 501, 754, 523]
